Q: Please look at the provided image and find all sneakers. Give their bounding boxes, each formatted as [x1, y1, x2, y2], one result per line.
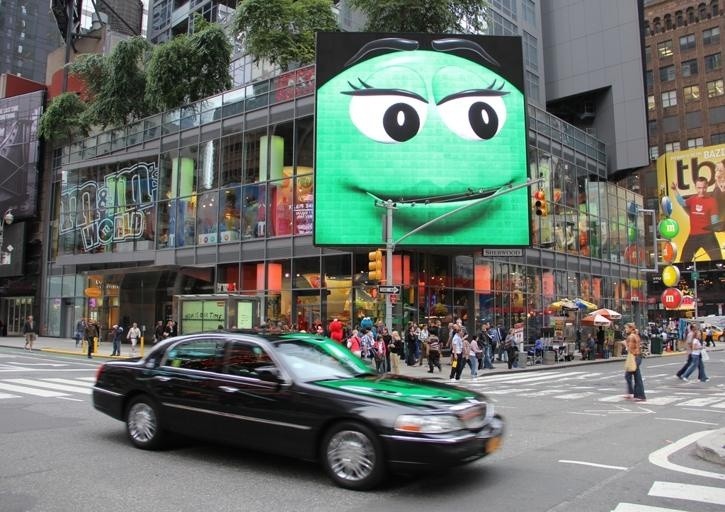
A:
[623, 394, 647, 404]
[676, 372, 711, 384]
[424, 362, 514, 385]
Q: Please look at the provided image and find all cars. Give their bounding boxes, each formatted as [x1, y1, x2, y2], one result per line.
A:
[91, 329, 507, 492]
[647, 315, 724, 342]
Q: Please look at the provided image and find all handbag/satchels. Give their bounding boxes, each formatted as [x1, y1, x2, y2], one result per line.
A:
[701, 347, 710, 363]
[624, 352, 638, 373]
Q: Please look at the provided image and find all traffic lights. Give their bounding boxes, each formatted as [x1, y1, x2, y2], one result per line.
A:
[536, 191, 544, 215]
[368, 251, 377, 280]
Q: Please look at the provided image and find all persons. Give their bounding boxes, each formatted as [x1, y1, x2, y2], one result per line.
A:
[671, 176, 722, 263]
[623, 322, 646, 402]
[75, 316, 178, 359]
[253, 317, 519, 381]
[579, 333, 594, 360]
[707, 326, 715, 347]
[23, 315, 36, 352]
[595, 326, 605, 353]
[704, 327, 710, 345]
[217, 324, 223, 329]
[676, 323, 709, 380]
[709, 160, 725, 233]
[681, 330, 710, 382]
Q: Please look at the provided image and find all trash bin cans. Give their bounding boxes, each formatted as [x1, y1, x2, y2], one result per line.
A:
[518, 352, 528, 368]
[651, 338, 663, 354]
[543, 351, 556, 365]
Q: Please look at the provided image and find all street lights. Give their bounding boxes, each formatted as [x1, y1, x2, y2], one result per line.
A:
[0, 207, 13, 246]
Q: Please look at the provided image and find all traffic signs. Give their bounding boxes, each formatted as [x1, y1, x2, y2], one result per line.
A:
[379, 285, 400, 293]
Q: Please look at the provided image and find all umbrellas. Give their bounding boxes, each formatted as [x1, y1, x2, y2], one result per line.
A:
[548, 297, 622, 327]
